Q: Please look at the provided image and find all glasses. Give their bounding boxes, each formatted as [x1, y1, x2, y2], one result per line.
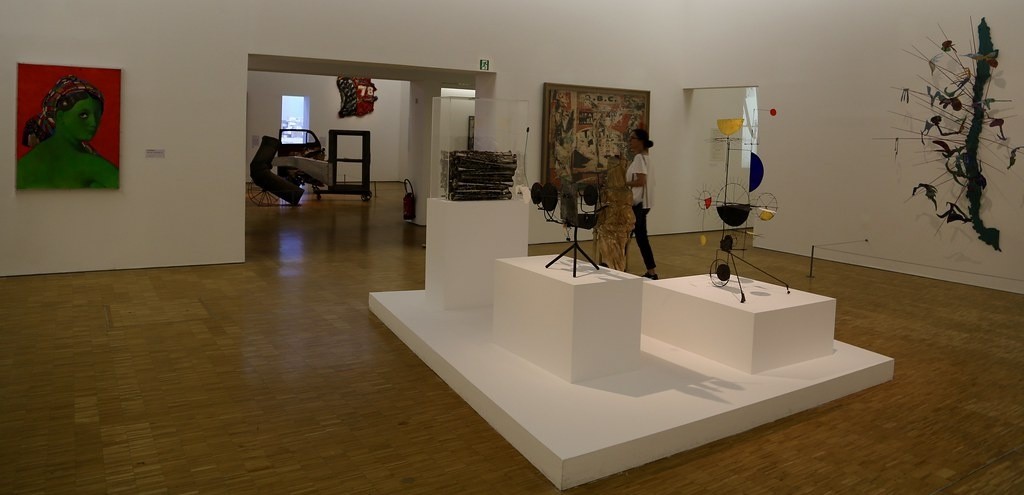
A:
[630, 136, 638, 139]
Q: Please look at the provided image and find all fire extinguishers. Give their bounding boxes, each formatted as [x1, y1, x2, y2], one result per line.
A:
[403, 179, 416, 220]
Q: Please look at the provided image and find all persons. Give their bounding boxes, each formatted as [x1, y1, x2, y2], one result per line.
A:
[624, 128, 659, 280]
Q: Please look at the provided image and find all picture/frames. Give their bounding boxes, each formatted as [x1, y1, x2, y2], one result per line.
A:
[541, 82, 651, 197]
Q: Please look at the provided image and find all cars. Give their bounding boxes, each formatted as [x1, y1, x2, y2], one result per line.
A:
[291, 126, 303, 138]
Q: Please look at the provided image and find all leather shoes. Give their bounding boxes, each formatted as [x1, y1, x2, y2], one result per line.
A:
[642, 272, 658, 280]
